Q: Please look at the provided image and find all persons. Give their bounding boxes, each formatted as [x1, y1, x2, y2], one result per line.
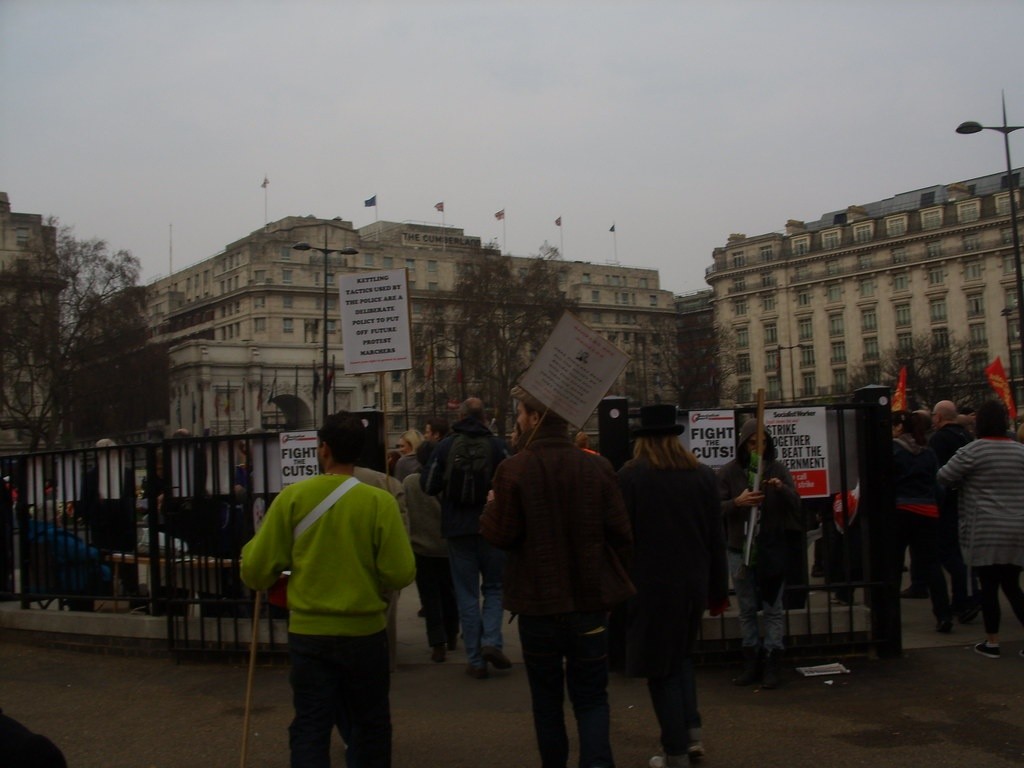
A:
[389, 416, 450, 482]
[403, 439, 459, 662]
[235, 427, 263, 495]
[715, 419, 801, 688]
[136, 428, 192, 523]
[479, 387, 638, 768]
[419, 398, 513, 679]
[576, 432, 600, 456]
[66, 438, 140, 598]
[239, 411, 416, 768]
[936, 398, 1024, 658]
[616, 404, 730, 768]
[29, 500, 111, 594]
[892, 400, 982, 633]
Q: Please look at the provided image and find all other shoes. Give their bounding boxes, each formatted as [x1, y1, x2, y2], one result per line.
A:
[688, 728, 705, 758]
[935, 621, 954, 633]
[901, 587, 930, 599]
[648, 751, 689, 768]
[829, 596, 855, 605]
[466, 666, 489, 679]
[481, 645, 512, 669]
[447, 639, 456, 650]
[432, 646, 446, 662]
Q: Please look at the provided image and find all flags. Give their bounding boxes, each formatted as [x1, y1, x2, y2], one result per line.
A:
[176, 364, 333, 427]
[364, 194, 615, 234]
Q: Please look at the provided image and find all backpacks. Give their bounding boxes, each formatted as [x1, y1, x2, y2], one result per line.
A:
[443, 433, 495, 506]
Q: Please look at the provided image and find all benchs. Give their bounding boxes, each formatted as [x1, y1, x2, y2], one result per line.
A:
[104, 551, 244, 615]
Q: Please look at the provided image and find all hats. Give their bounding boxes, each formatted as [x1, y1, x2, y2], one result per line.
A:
[510, 386, 554, 414]
[631, 404, 685, 439]
[95, 439, 116, 458]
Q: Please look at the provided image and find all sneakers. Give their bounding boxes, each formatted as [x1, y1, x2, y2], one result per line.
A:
[1018, 648, 1024, 656]
[974, 640, 1001, 659]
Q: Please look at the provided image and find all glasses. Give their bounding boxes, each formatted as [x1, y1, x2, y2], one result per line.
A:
[746, 440, 766, 446]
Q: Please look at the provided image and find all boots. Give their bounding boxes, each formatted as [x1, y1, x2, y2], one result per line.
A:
[732, 643, 763, 685]
[761, 648, 785, 688]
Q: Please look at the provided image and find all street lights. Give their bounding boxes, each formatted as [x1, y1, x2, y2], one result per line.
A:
[956, 88, 1024, 368]
[1000, 307, 1017, 431]
[777, 338, 805, 402]
[292, 226, 359, 424]
